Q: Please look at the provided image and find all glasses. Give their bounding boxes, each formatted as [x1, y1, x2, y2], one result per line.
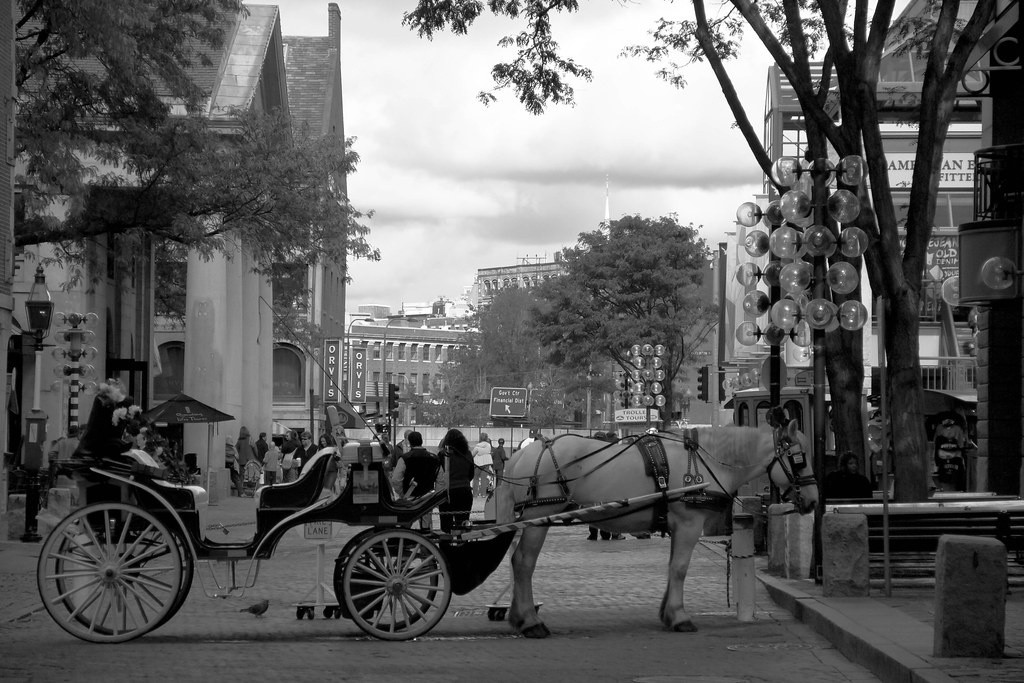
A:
[301, 438, 310, 441]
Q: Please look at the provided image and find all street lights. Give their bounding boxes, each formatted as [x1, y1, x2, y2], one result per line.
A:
[734, 197, 816, 552]
[344, 318, 375, 398]
[382, 318, 419, 443]
[768, 155, 870, 587]
[51, 310, 100, 439]
[626, 343, 666, 430]
[11, 260, 55, 474]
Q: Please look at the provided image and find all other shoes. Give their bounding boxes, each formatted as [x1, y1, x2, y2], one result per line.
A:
[600, 537, 603, 540]
[240, 494, 247, 497]
[618, 534, 625, 539]
[587, 536, 591, 540]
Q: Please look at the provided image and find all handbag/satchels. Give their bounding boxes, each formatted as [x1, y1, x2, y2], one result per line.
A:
[435, 446, 450, 504]
[282, 447, 297, 470]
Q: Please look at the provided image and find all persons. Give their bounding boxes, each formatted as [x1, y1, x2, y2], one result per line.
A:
[316, 434, 344, 490]
[293, 431, 318, 478]
[262, 441, 283, 485]
[391, 429, 413, 467]
[392, 432, 446, 563]
[471, 432, 493, 498]
[586, 432, 626, 540]
[438, 429, 475, 533]
[382, 433, 393, 451]
[235, 426, 258, 484]
[517, 426, 538, 451]
[492, 438, 509, 488]
[225, 436, 247, 497]
[372, 422, 384, 439]
[336, 425, 348, 442]
[834, 452, 874, 498]
[281, 430, 301, 483]
[255, 432, 269, 465]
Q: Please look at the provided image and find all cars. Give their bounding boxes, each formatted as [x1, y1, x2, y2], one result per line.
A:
[722, 386, 978, 502]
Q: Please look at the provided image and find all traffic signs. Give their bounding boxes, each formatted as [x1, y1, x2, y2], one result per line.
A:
[489, 387, 527, 417]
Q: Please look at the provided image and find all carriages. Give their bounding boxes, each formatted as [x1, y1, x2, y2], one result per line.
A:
[31, 408, 822, 642]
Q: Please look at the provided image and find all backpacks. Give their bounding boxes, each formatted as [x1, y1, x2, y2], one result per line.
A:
[225, 444, 236, 462]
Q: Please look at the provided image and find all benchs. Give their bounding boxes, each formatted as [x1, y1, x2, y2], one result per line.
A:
[253, 447, 335, 507]
[326, 405, 388, 464]
[117, 447, 209, 503]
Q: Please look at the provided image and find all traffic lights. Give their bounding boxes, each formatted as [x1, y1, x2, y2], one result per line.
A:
[698, 366, 709, 403]
[374, 424, 384, 433]
[389, 384, 400, 410]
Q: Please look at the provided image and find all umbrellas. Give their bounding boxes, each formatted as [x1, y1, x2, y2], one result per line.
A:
[141, 392, 236, 423]
